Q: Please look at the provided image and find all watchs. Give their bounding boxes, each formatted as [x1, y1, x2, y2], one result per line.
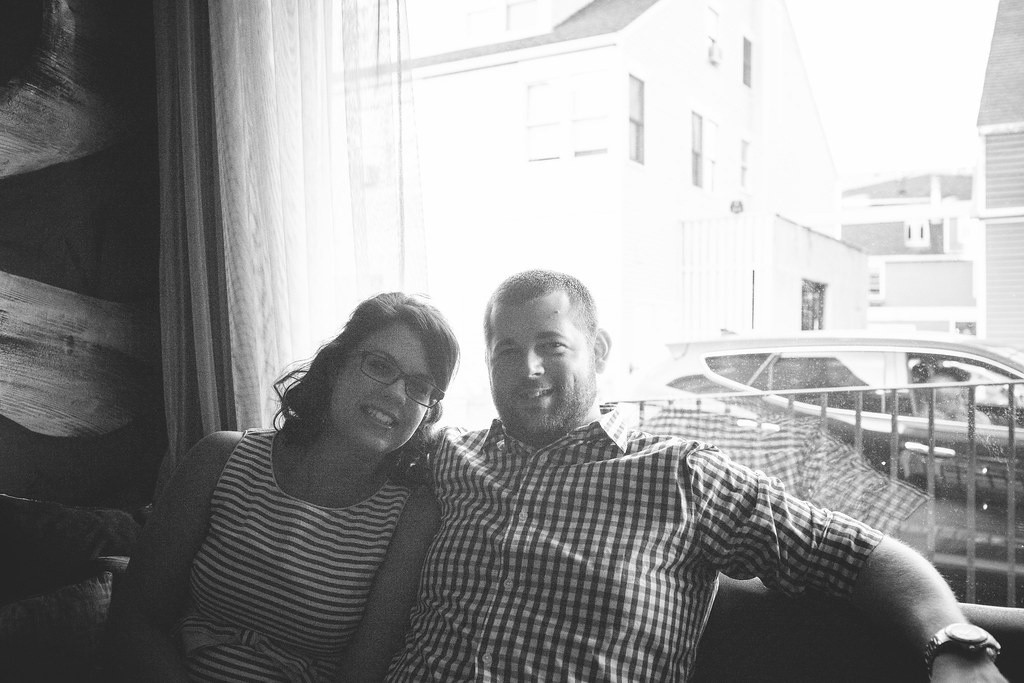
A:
[922, 622, 1002, 683]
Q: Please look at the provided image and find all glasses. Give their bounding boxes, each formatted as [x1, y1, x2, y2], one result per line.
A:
[359, 343, 445, 407]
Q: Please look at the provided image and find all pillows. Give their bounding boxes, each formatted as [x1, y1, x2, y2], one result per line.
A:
[1, 572, 116, 683]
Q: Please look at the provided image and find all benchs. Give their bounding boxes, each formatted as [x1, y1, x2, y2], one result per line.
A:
[98, 556, 1024, 681]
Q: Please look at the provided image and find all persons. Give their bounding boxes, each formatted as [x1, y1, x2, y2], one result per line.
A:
[916, 367, 990, 424]
[383, 276, 1009, 683]
[117, 301, 454, 683]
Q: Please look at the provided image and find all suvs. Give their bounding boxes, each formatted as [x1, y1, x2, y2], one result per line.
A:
[645, 331, 1024, 566]
